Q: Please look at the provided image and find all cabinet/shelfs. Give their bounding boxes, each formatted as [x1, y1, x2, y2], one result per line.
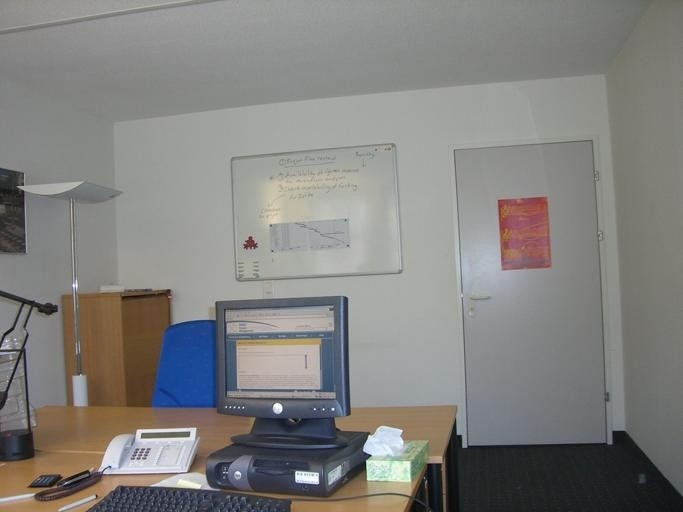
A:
[58, 290, 172, 408]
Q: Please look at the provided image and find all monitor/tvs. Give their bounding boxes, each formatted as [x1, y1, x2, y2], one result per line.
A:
[215, 295, 351, 450]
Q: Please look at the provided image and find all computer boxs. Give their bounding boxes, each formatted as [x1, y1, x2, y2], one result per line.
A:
[206, 431, 371, 497]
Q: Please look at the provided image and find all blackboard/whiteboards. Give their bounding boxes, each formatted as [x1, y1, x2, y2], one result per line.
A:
[231, 143, 403, 282]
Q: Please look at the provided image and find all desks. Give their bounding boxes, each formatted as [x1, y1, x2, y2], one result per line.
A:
[17, 402, 461, 508]
[0, 452, 426, 512]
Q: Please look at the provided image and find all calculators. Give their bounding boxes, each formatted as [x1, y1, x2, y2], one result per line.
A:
[28, 475, 62, 487]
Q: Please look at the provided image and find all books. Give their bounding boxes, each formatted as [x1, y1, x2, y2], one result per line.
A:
[99, 281, 151, 294]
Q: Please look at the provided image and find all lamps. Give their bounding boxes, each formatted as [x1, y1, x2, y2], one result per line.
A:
[13, 178, 125, 407]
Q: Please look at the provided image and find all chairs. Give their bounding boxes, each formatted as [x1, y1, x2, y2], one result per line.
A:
[149, 321, 219, 407]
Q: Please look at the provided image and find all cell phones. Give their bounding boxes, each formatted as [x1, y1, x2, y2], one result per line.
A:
[57, 469, 90, 487]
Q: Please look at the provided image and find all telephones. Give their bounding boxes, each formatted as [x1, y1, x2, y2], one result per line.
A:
[97, 427, 201, 475]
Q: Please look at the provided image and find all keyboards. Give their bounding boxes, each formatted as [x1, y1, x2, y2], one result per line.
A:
[85, 484, 291, 512]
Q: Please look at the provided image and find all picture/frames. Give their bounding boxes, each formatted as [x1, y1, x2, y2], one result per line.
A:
[0, 167, 29, 255]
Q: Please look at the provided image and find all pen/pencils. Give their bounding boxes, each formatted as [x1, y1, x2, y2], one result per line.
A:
[58, 494, 97, 512]
